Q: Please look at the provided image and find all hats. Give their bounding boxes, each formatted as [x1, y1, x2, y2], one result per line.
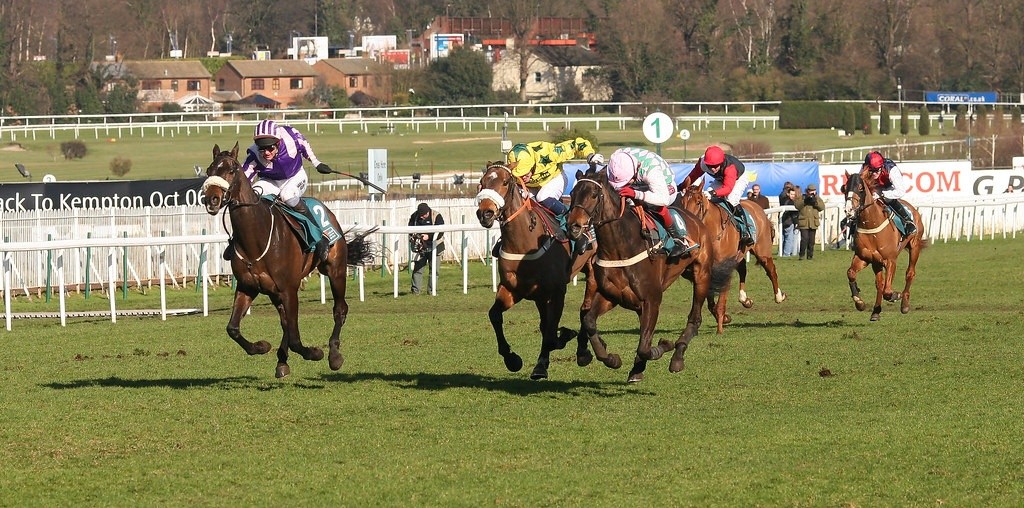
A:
[746, 189, 754, 194]
[418, 203, 429, 215]
[807, 184, 816, 190]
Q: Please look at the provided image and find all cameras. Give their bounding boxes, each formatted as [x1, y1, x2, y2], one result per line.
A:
[810, 193, 815, 198]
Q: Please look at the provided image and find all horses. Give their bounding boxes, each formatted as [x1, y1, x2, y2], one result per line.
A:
[844, 167, 929, 322]
[203, 141, 379, 378]
[475, 158, 787, 385]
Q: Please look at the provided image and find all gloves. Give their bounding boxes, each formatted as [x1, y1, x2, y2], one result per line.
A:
[316, 163, 332, 174]
[617, 187, 635, 198]
[704, 191, 712, 200]
[804, 197, 816, 206]
[872, 192, 882, 201]
[587, 153, 604, 166]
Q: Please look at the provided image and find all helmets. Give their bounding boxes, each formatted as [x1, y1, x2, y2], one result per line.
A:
[703, 146, 725, 165]
[508, 143, 535, 177]
[607, 152, 638, 187]
[253, 120, 281, 146]
[865, 152, 883, 169]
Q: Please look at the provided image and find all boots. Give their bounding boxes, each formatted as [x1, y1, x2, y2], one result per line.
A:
[490, 204, 755, 259]
[223, 245, 233, 260]
[897, 205, 916, 234]
[292, 198, 334, 264]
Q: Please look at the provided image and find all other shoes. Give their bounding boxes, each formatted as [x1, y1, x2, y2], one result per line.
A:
[799, 256, 804, 260]
[413, 291, 419, 295]
[807, 255, 812, 259]
[427, 291, 432, 295]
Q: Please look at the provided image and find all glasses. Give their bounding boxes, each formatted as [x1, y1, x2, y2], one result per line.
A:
[258, 145, 275, 152]
[706, 164, 720, 168]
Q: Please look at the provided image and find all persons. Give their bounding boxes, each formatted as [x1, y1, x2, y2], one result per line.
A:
[746, 182, 825, 260]
[223, 119, 332, 261]
[490, 137, 603, 257]
[938, 114, 974, 128]
[841, 152, 917, 235]
[677, 146, 754, 246]
[607, 147, 690, 258]
[407, 203, 446, 295]
